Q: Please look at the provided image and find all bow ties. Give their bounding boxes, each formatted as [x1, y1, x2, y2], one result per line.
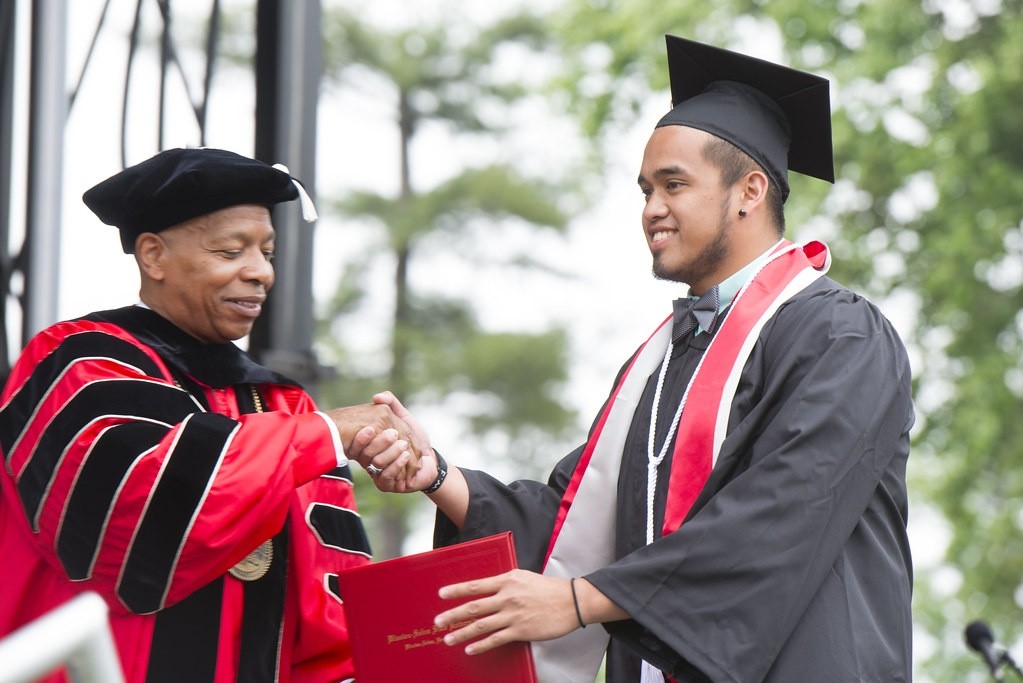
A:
[672, 285, 719, 345]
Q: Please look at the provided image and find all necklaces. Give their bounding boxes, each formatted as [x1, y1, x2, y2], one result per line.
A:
[176, 380, 272, 580]
[647, 241, 802, 544]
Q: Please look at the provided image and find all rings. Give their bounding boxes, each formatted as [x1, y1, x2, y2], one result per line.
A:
[366, 464, 382, 476]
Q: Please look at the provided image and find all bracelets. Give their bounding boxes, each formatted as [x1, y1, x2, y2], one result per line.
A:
[422, 448, 448, 494]
[571, 578, 586, 629]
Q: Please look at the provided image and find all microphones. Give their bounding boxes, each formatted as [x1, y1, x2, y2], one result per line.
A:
[964, 621, 1005, 681]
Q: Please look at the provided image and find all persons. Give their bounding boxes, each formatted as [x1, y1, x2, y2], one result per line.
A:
[348, 33, 915, 683]
[0, 147, 419, 683]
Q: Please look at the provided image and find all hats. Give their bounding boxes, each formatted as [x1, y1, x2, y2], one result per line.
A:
[655, 34, 835, 204]
[83, 148, 317, 254]
